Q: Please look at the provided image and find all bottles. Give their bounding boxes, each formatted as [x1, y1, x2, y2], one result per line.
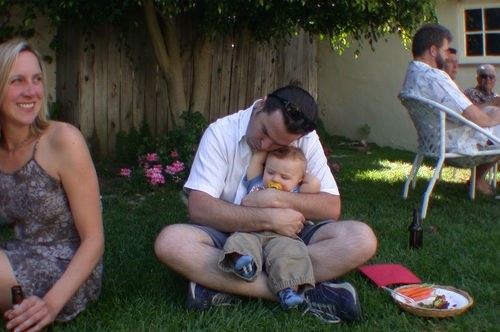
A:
[407, 208, 423, 251]
[11, 285, 27, 309]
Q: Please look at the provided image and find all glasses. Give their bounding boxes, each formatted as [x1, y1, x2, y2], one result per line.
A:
[268, 93, 316, 132]
[479, 74, 496, 79]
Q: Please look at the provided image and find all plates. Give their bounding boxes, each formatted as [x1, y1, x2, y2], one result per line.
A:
[391, 283, 473, 317]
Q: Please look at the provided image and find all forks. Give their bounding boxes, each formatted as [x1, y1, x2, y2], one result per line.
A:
[382, 286, 421, 307]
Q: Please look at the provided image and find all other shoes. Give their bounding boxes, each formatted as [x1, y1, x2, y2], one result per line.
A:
[466, 175, 494, 195]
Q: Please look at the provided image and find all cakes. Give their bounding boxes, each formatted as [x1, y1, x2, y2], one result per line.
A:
[417, 294, 450, 309]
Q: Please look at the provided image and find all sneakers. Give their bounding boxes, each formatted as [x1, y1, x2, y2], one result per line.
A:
[298, 282, 361, 323]
[190, 281, 240, 311]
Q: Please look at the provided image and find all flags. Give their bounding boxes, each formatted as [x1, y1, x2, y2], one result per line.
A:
[360, 263, 422, 288]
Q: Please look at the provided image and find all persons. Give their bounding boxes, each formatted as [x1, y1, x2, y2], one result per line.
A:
[155, 85, 378, 324]
[403, 23, 500, 200]
[462, 64, 500, 108]
[0, 39, 105, 332]
[438, 47, 500, 193]
[217, 145, 321, 309]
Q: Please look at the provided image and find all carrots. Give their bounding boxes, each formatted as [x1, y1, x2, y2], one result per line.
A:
[395, 285, 436, 303]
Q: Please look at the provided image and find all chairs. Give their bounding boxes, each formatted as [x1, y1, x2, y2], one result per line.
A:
[397, 92, 500, 218]
